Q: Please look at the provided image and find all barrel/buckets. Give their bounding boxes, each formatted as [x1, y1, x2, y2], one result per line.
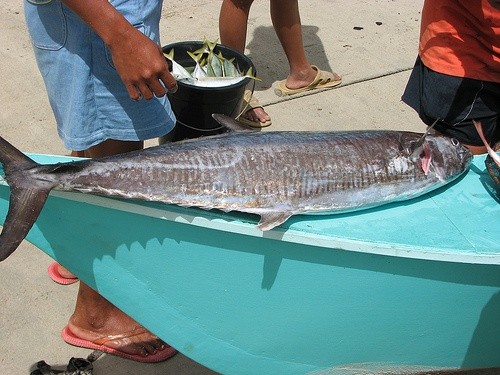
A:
[160, 41, 257, 142]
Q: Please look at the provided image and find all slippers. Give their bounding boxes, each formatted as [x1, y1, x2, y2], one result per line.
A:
[62, 323, 179, 363]
[48, 261, 79, 285]
[235, 85, 271, 127]
[272, 65, 342, 97]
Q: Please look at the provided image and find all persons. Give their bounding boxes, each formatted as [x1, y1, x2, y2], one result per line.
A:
[23, 0, 179, 362]
[219, 0, 342, 127]
[399, 0, 500, 156]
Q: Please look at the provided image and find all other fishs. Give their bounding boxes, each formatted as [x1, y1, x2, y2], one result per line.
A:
[162, 35, 263, 88]
[0, 130, 475, 262]
